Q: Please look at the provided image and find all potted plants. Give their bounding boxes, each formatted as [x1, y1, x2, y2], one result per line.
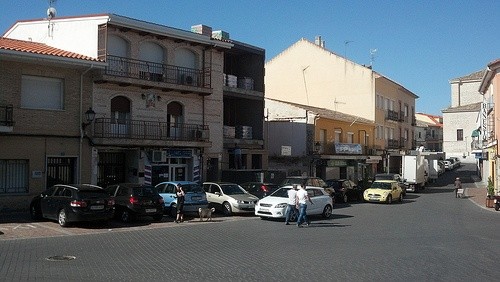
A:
[486, 176, 496, 207]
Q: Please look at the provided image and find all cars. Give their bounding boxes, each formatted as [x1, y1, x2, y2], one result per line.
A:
[443, 157, 461, 171]
[363, 180, 403, 204]
[254, 186, 333, 222]
[202, 182, 259, 216]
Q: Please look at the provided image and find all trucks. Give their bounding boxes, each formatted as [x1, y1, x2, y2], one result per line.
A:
[409, 151, 447, 185]
[388, 155, 425, 193]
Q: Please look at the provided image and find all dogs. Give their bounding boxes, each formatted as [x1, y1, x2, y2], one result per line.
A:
[197, 207, 215, 222]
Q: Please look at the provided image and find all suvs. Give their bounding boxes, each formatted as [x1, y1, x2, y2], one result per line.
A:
[155, 181, 209, 218]
[30, 184, 115, 227]
[240, 173, 407, 203]
[105, 183, 165, 222]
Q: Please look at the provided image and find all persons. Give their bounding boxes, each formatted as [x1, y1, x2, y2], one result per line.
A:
[453, 177, 463, 199]
[295, 183, 313, 227]
[172, 183, 186, 222]
[284, 184, 305, 225]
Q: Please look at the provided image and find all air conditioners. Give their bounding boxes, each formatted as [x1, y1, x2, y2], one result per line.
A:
[183, 74, 194, 86]
[149, 151, 166, 162]
[192, 130, 205, 140]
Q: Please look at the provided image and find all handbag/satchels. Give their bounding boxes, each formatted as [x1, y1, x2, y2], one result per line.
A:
[457, 188, 464, 194]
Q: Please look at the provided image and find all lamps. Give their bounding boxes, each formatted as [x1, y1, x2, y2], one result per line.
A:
[313, 141, 320, 153]
[82, 107, 97, 130]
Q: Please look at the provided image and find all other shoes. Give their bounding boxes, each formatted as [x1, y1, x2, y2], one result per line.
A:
[307, 222, 310, 226]
[285, 222, 289, 225]
[297, 225, 302, 227]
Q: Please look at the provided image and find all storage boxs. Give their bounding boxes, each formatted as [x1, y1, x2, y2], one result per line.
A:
[224, 73, 255, 91]
[223, 126, 253, 139]
